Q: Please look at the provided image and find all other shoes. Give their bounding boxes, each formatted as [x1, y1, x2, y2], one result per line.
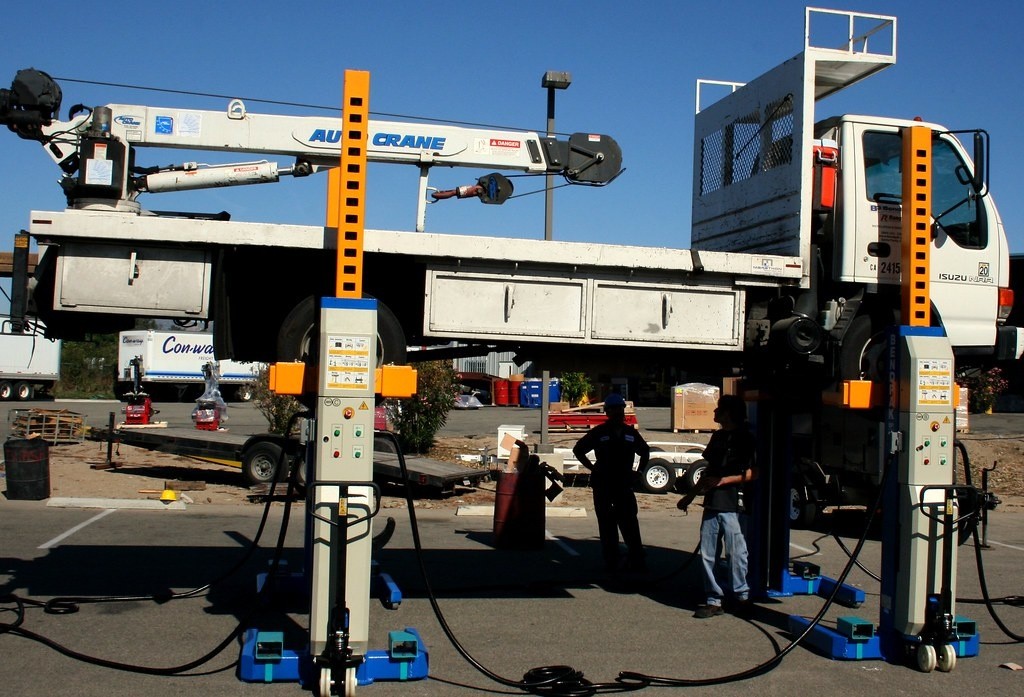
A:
[736, 598, 757, 616]
[608, 573, 616, 584]
[695, 605, 723, 618]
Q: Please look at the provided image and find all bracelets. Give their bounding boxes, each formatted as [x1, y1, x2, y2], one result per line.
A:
[638, 470, 643, 474]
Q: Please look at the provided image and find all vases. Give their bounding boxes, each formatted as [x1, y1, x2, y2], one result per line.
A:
[984, 404, 993, 414]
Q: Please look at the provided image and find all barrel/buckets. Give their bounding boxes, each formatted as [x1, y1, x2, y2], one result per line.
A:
[3, 439, 51, 501]
[495, 380, 520, 404]
[492, 471, 545, 548]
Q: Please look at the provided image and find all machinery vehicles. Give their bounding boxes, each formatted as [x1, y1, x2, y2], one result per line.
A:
[0, 6, 1015, 412]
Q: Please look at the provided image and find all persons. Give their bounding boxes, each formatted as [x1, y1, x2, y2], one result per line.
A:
[677, 395, 760, 619]
[573, 393, 650, 568]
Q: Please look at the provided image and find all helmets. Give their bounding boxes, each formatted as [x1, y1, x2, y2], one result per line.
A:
[603, 393, 626, 410]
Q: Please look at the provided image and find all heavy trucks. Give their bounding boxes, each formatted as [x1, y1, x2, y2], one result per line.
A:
[115, 329, 274, 402]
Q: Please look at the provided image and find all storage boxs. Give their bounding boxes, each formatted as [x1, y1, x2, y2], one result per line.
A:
[669, 386, 721, 431]
[549, 401, 570, 412]
[509, 374, 524, 382]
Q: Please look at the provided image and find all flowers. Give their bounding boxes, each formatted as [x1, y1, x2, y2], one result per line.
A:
[972, 366, 1009, 410]
